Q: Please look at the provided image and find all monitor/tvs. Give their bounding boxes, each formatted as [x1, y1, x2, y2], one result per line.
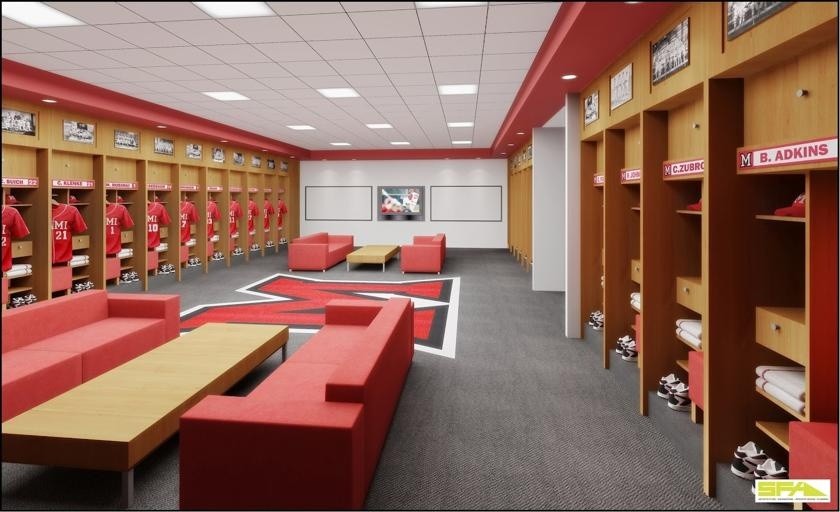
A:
[380, 186, 424, 217]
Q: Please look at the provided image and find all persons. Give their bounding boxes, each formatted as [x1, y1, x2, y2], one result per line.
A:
[382, 188, 420, 213]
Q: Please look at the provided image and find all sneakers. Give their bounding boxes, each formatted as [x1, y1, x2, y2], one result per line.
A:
[751, 457, 788, 498]
[613, 333, 636, 354]
[592, 314, 604, 331]
[654, 373, 683, 399]
[589, 310, 603, 326]
[620, 340, 639, 362]
[665, 382, 693, 413]
[727, 440, 769, 480]
[6, 237, 290, 309]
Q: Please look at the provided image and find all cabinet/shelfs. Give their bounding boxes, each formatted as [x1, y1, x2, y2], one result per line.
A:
[2, 142, 290, 310]
[578, 12, 839, 509]
[506, 164, 533, 272]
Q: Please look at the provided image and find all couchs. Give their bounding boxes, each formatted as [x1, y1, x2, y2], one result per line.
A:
[0, 288, 184, 428]
[396, 232, 447, 277]
[285, 228, 356, 275]
[175, 292, 417, 509]
